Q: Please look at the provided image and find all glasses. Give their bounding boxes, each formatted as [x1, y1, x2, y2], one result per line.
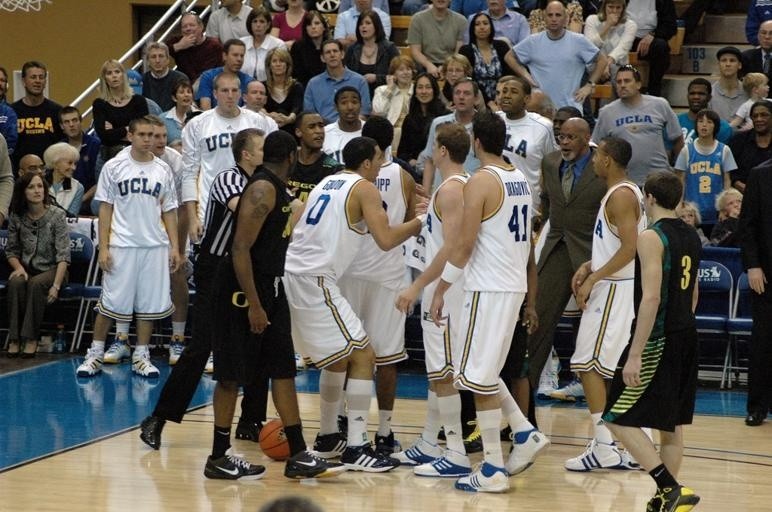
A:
[556, 133, 578, 140]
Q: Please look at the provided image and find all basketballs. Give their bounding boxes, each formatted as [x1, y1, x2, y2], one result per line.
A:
[259, 420, 291, 461]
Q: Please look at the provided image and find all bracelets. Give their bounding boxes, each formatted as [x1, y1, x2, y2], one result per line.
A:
[441, 263, 463, 288]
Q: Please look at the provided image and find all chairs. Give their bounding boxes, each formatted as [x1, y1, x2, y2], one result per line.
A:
[0, 227, 12, 349]
[693, 257, 731, 392]
[49, 232, 95, 353]
[75, 246, 101, 356]
[721, 271, 754, 389]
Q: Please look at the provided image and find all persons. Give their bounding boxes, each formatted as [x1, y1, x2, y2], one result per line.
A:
[429, 113, 549, 493]
[600, 172, 700, 512]
[264, 0, 396, 182]
[464, 157, 530, 454]
[504, 1, 701, 177]
[497, 117, 617, 443]
[0, 61, 104, 358]
[75, 111, 429, 486]
[564, 135, 646, 471]
[665, 1, 769, 387]
[94, 0, 276, 175]
[373, 1, 553, 171]
[392, 121, 472, 476]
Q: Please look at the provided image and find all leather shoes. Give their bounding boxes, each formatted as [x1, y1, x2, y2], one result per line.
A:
[745, 411, 767, 424]
[140, 416, 167, 450]
[236, 418, 263, 442]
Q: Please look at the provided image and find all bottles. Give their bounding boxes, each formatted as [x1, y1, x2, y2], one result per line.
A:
[53, 324, 68, 354]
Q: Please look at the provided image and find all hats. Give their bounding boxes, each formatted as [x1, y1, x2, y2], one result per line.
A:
[717, 46, 742, 60]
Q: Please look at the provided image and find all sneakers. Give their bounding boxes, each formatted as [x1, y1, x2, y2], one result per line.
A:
[285, 415, 402, 479]
[295, 353, 308, 371]
[537, 371, 585, 401]
[204, 351, 215, 373]
[76, 334, 185, 377]
[389, 419, 551, 492]
[565, 439, 644, 470]
[204, 446, 266, 480]
[646, 485, 700, 512]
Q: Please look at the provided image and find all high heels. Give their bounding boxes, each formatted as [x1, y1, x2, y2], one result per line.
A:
[21, 338, 38, 358]
[7, 341, 20, 359]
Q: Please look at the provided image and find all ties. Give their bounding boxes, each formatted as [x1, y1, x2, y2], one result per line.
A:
[561, 164, 573, 205]
